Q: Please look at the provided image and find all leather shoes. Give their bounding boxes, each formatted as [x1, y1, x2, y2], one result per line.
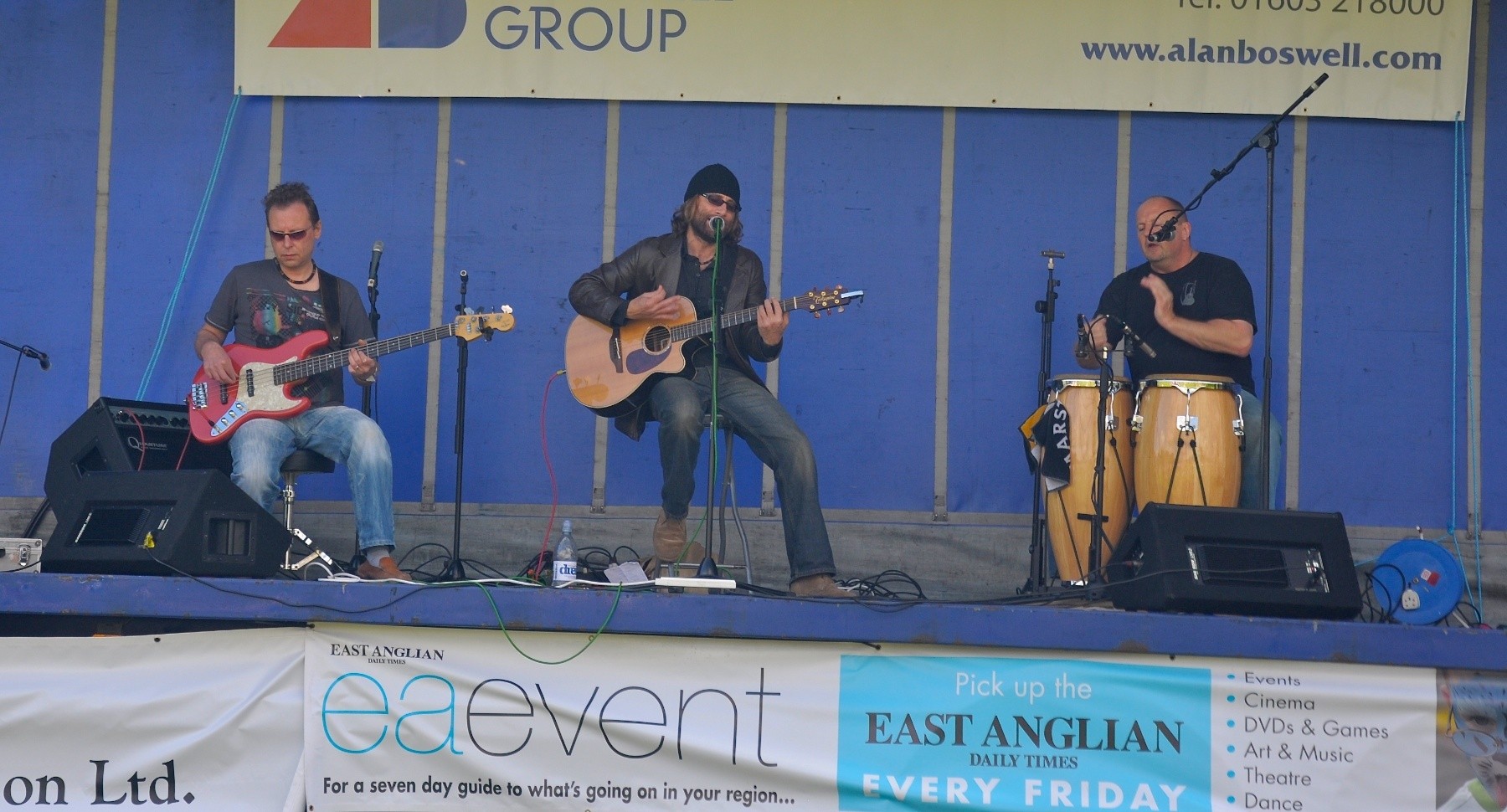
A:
[355, 556, 412, 582]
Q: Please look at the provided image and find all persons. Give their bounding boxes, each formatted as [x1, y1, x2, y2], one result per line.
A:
[1074, 196, 1281, 510]
[194, 179, 411, 582]
[568, 163, 856, 596]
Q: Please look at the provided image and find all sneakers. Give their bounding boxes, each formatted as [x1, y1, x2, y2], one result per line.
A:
[653, 507, 686, 561]
[790, 573, 857, 599]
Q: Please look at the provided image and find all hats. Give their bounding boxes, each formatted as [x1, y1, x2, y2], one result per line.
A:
[684, 164, 741, 209]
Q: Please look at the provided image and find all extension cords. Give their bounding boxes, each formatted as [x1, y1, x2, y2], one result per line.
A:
[655, 577, 737, 589]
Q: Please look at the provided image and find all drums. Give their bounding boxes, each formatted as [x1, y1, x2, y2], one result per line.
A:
[1041, 374, 1137, 588]
[1133, 373, 1243, 516]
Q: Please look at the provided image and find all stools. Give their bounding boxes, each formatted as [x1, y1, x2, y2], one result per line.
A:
[646, 411, 753, 593]
[280, 448, 349, 579]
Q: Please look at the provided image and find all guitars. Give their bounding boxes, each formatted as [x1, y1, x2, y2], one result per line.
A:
[564, 282, 865, 418]
[187, 304, 515, 444]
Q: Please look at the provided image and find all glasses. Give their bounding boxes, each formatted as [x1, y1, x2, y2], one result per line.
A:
[698, 193, 741, 215]
[267, 223, 314, 242]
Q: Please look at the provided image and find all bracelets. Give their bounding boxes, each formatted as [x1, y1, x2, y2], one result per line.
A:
[366, 366, 377, 382]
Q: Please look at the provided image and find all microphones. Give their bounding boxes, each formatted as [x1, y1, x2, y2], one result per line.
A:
[709, 216, 725, 231]
[1113, 318, 1157, 359]
[39, 353, 52, 370]
[1076, 316, 1091, 358]
[1149, 231, 1175, 242]
[367, 240, 385, 293]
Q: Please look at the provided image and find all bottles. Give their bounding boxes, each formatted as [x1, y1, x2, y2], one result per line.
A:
[551, 520, 577, 587]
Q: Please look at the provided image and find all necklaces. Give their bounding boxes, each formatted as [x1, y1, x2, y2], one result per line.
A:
[274, 257, 316, 285]
[700, 257, 714, 265]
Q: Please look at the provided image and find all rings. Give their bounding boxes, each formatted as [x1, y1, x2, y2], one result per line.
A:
[356, 365, 359, 370]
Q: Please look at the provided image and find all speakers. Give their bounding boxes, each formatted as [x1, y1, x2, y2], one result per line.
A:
[44, 397, 235, 523]
[39, 468, 293, 580]
[1107, 501, 1364, 621]
